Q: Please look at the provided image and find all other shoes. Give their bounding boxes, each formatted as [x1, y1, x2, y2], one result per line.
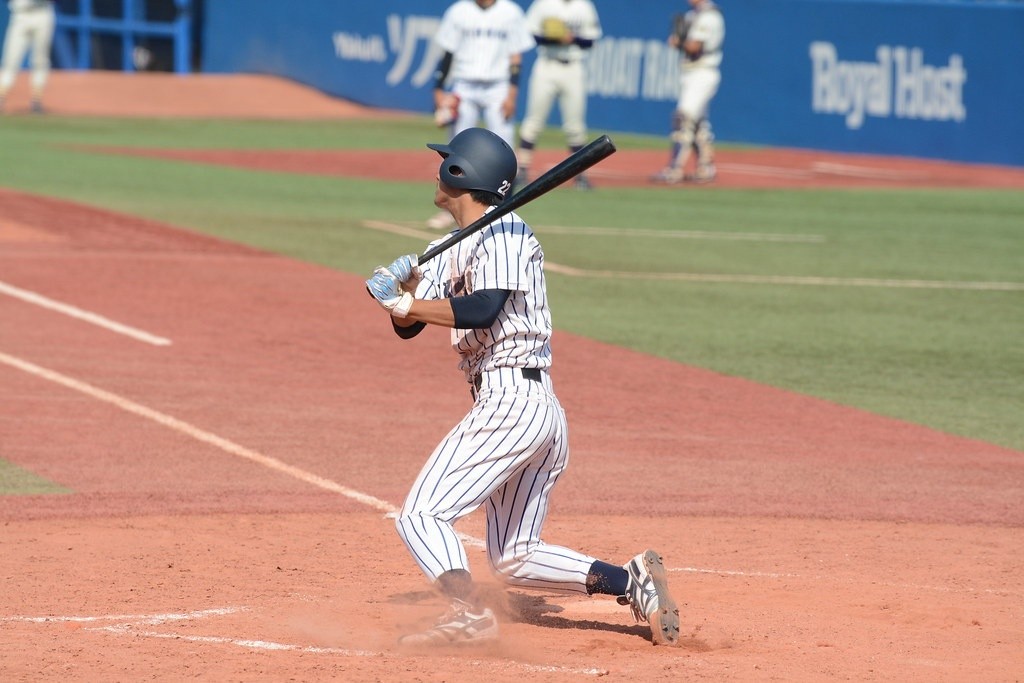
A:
[687, 171, 714, 181]
[575, 176, 590, 189]
[649, 168, 683, 183]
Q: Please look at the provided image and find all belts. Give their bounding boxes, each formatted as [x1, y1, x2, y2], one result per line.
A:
[470, 369, 540, 403]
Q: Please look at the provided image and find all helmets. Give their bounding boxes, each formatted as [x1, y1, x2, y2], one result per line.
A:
[427, 126, 517, 198]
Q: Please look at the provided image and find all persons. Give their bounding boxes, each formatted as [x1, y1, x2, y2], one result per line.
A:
[0, 0, 56, 113]
[516, 0, 602, 190]
[432, 0, 537, 152]
[649, 0, 726, 184]
[366, 127, 682, 645]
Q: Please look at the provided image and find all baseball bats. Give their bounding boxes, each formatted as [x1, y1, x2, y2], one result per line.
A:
[364, 133, 617, 299]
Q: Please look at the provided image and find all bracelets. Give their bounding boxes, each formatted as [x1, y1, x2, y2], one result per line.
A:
[510, 64, 523, 87]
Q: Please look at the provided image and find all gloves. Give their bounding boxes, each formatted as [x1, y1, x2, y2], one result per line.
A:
[388, 255, 418, 283]
[366, 266, 414, 318]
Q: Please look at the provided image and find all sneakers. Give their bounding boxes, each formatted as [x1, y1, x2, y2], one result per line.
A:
[397, 599, 498, 645]
[617, 550, 679, 645]
